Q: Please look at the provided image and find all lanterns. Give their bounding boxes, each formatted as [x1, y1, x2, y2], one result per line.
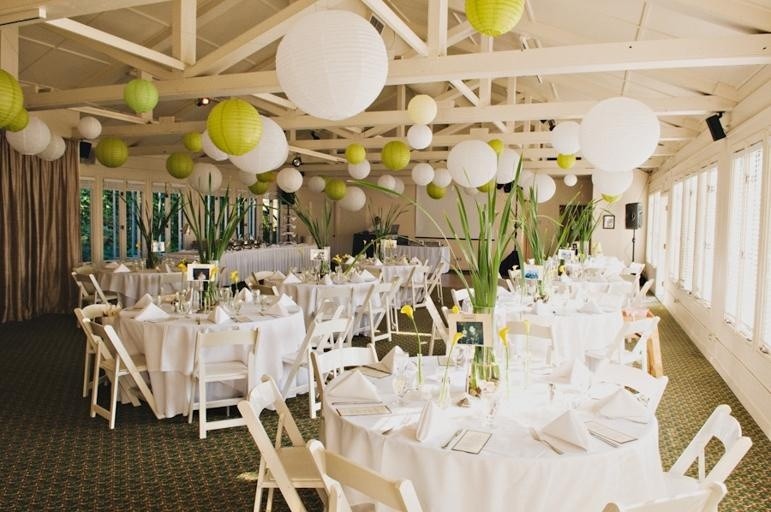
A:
[79, 116, 128, 168]
[0, 69, 65, 163]
[123, 79, 159, 112]
[166, 96, 302, 195]
[407, 94, 438, 149]
[445, 137, 521, 193]
[309, 144, 371, 211]
[463, 0, 527, 38]
[551, 96, 659, 202]
[517, 170, 555, 202]
[412, 163, 452, 199]
[376, 140, 409, 196]
[273, 7, 391, 122]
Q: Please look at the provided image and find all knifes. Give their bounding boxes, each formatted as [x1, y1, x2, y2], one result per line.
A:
[442, 427, 464, 449]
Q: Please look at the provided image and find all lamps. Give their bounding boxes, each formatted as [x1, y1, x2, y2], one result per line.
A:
[706, 111, 728, 142]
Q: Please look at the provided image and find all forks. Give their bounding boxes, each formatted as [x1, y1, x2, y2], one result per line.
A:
[195, 316, 201, 326]
[528, 426, 563, 454]
[383, 415, 411, 434]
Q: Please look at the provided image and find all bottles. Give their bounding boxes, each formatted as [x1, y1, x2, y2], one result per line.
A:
[174, 290, 192, 314]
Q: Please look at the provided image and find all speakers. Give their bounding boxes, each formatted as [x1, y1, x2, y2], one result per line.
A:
[625, 202, 642, 230]
[280, 191, 296, 205]
[705, 114, 726, 142]
[78, 140, 92, 159]
